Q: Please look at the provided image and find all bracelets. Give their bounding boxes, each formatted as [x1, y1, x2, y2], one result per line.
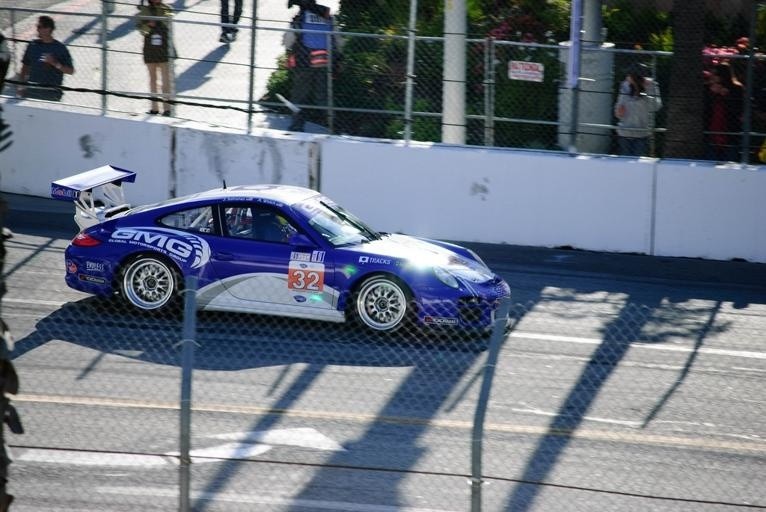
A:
[56, 62, 63, 69]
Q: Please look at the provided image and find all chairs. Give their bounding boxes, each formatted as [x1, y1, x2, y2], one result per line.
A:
[254, 216, 288, 242]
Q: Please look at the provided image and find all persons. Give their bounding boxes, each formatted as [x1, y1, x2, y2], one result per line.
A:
[614, 63, 664, 156]
[266, 212, 297, 244]
[283, 0, 344, 135]
[698, 63, 745, 161]
[134, 1, 178, 116]
[0, 29, 11, 92]
[16, 14, 75, 102]
[219, 1, 243, 45]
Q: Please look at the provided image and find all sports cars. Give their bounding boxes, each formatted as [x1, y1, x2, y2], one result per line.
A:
[50, 163, 512, 341]
